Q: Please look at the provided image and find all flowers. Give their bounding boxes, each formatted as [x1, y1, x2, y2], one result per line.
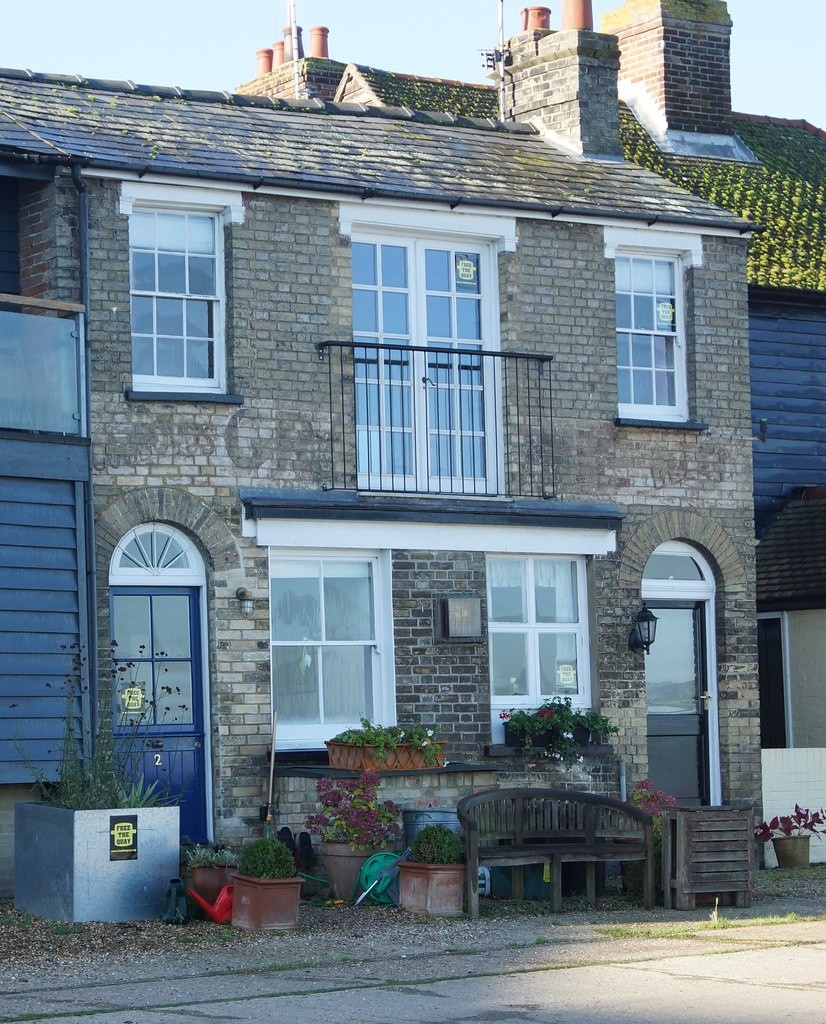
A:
[305, 773, 402, 849]
[626, 777, 678, 845]
[494, 696, 621, 768]
[755, 807, 826, 842]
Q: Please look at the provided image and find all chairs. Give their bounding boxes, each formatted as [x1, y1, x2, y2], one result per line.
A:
[458, 789, 659, 922]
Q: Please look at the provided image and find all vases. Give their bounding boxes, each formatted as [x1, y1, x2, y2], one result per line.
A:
[568, 720, 589, 747]
[771, 834, 811, 868]
[321, 840, 396, 904]
[530, 720, 552, 747]
[403, 807, 461, 846]
[502, 722, 531, 747]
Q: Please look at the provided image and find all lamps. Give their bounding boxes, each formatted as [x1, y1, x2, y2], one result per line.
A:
[628, 600, 660, 659]
[235, 587, 255, 618]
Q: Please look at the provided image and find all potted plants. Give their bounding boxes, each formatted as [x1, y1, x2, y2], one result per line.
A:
[398, 827, 466, 917]
[232, 838, 305, 931]
[324, 708, 447, 773]
[7, 638, 201, 922]
[185, 847, 237, 900]
